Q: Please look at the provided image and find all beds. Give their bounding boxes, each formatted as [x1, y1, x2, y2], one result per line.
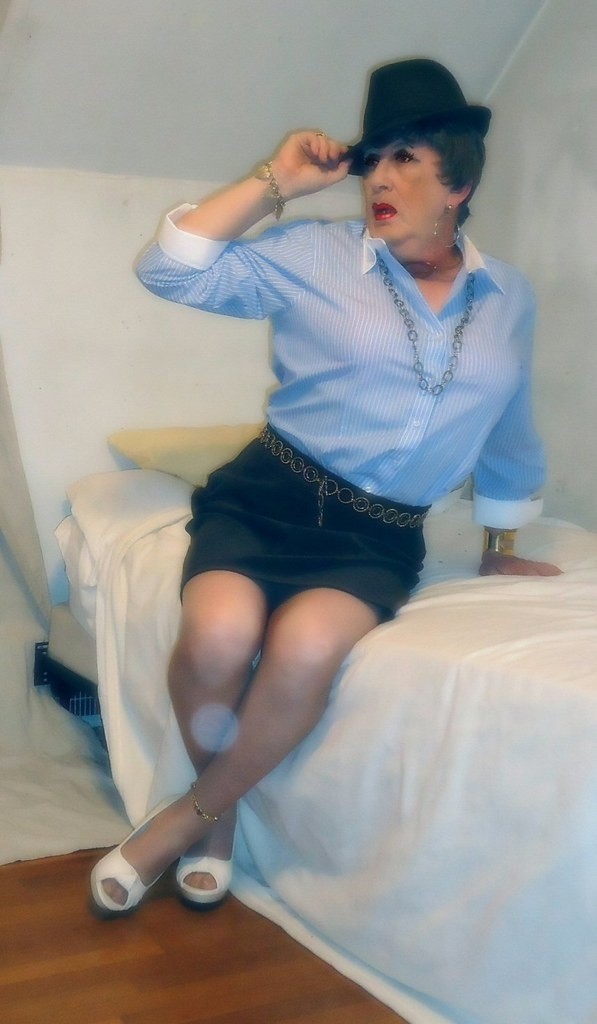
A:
[0, 164, 597, 1023]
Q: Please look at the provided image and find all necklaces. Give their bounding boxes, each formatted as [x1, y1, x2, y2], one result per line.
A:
[375, 253, 474, 394]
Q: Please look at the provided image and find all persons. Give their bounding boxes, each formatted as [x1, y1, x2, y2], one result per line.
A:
[88, 58, 566, 921]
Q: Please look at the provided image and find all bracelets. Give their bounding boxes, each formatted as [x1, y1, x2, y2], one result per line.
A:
[257, 161, 286, 221]
[482, 528, 518, 556]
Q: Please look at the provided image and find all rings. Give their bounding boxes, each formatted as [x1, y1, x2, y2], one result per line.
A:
[315, 131, 324, 136]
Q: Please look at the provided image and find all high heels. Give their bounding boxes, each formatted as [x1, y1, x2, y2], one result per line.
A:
[174, 800, 239, 905]
[89, 794, 184, 913]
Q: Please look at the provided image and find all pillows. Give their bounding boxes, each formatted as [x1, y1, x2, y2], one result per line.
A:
[105, 423, 261, 488]
[64, 469, 193, 564]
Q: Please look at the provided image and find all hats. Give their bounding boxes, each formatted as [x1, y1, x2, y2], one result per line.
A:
[339, 58, 492, 176]
